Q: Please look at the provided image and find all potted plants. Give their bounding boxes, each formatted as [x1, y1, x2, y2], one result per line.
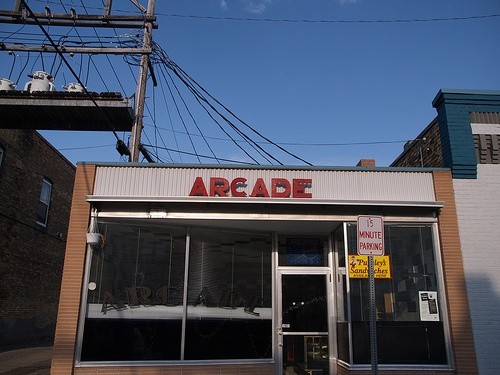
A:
[85, 231, 105, 248]
